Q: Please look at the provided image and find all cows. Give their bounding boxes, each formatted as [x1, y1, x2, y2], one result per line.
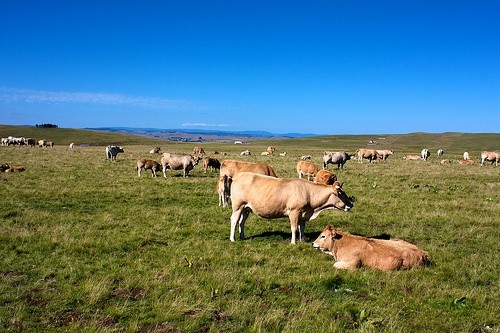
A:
[229, 170, 353, 244]
[38, 140, 54, 149]
[240, 147, 287, 156]
[479, 150, 500, 166]
[440, 159, 453, 164]
[1, 136, 36, 148]
[159, 152, 203, 178]
[0, 162, 10, 173]
[323, 151, 352, 170]
[136, 159, 168, 177]
[456, 158, 474, 165]
[192, 147, 206, 156]
[402, 154, 420, 160]
[203, 157, 221, 172]
[69, 142, 75, 149]
[149, 147, 160, 153]
[312, 223, 428, 271]
[105, 145, 124, 161]
[420, 148, 430, 161]
[217, 160, 278, 209]
[357, 149, 393, 164]
[436, 149, 443, 157]
